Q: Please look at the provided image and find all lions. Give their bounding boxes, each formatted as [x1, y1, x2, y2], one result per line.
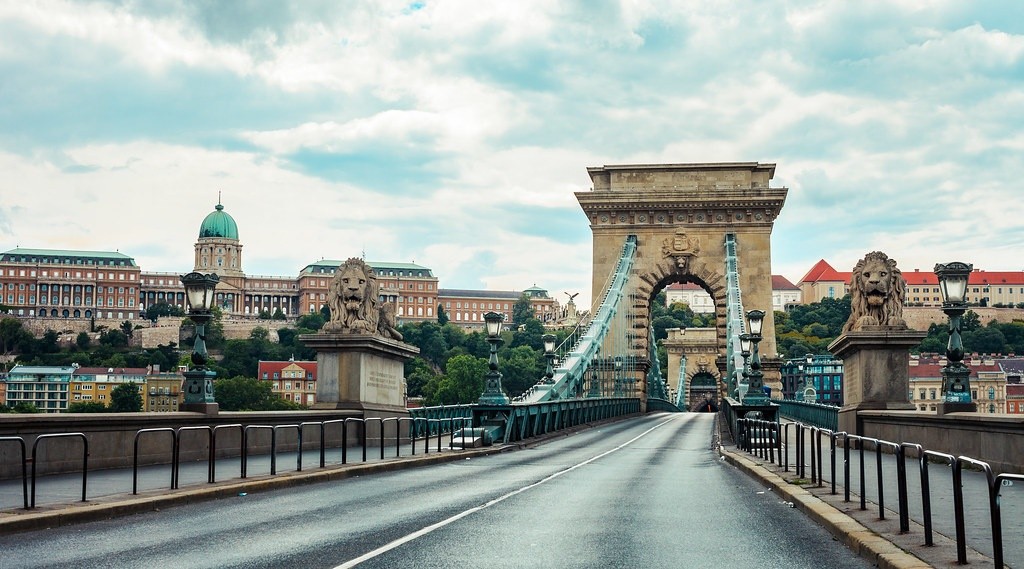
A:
[322, 257, 403, 340]
[841, 251, 908, 334]
